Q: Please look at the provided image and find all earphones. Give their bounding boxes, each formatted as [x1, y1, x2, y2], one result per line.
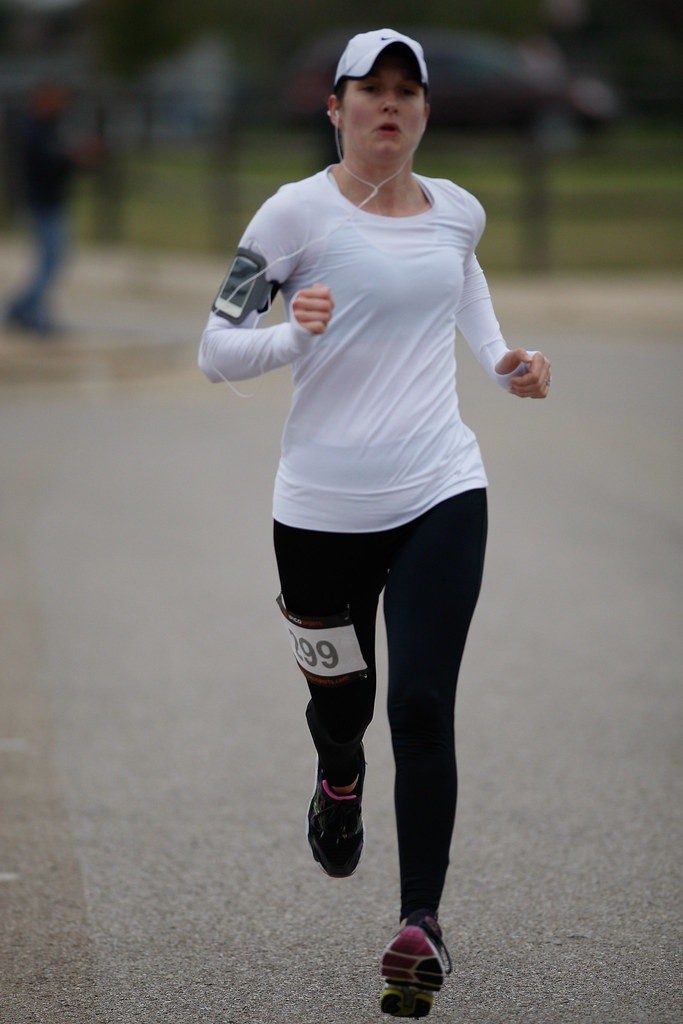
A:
[421, 119, 427, 134]
[334, 110, 341, 120]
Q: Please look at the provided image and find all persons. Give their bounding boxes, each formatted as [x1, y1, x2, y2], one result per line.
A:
[197, 29, 551, 1018]
[6, 80, 104, 334]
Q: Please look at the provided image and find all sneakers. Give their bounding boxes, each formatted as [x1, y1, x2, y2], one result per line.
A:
[381, 911, 453, 1017]
[307, 742, 366, 879]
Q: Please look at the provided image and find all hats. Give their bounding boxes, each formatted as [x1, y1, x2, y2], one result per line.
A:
[334, 28, 429, 100]
